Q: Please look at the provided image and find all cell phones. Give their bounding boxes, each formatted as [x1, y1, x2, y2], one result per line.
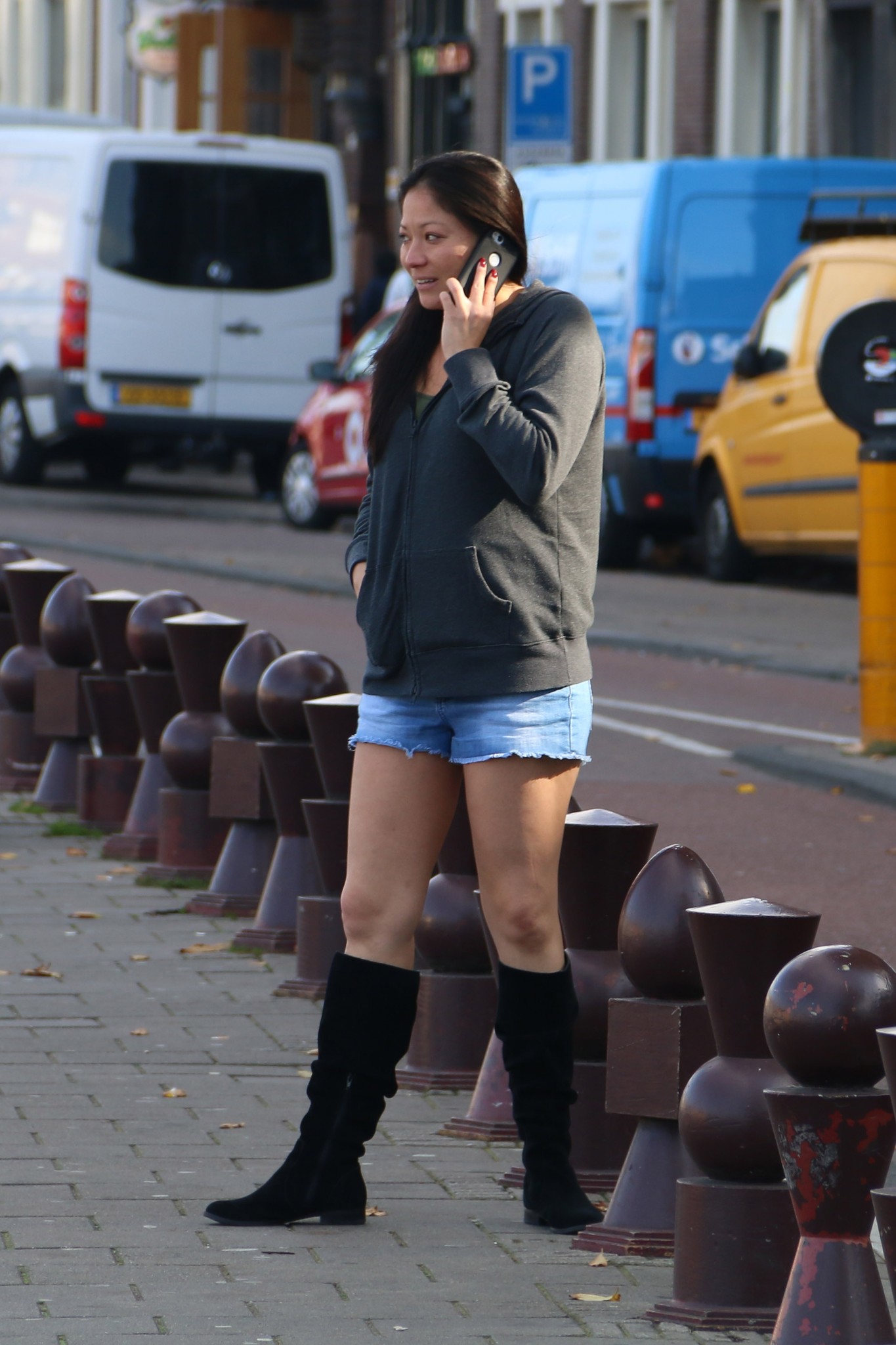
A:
[446, 226, 521, 300]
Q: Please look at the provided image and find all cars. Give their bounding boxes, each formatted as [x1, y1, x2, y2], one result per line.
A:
[278, 299, 410, 529]
[689, 216, 894, 583]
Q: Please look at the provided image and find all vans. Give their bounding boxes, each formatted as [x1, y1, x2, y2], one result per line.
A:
[1, 108, 346, 487]
[512, 154, 895, 556]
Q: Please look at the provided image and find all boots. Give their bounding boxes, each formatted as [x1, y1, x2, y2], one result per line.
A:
[494, 954, 607, 1236]
[204, 952, 423, 1227]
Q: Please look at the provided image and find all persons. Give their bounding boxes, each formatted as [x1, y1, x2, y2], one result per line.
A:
[202, 150, 605, 1236]
